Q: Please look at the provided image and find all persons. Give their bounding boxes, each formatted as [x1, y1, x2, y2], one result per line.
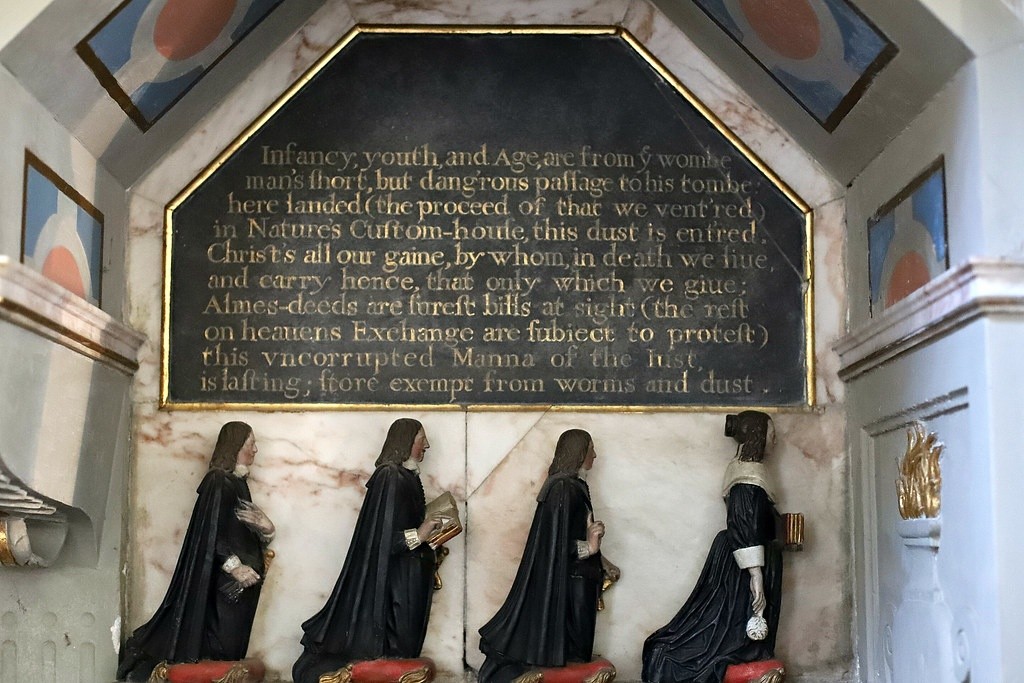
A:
[641, 410, 786, 683]
[291, 418, 442, 683]
[116, 422, 276, 683]
[477, 429, 605, 683]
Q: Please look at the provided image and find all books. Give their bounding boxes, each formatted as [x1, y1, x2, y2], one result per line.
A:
[425, 491, 463, 551]
